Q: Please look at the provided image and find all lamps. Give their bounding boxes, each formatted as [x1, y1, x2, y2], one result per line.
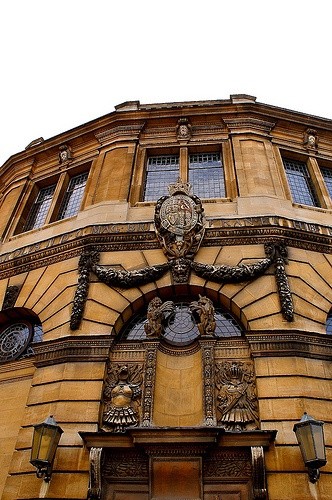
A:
[29, 414, 64, 485]
[292, 411, 328, 485]
